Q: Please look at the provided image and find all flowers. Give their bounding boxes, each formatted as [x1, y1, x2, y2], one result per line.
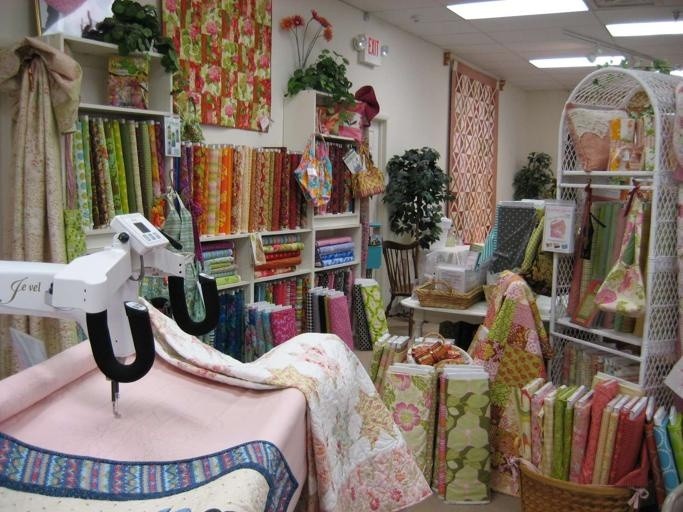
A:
[277, 7, 336, 67]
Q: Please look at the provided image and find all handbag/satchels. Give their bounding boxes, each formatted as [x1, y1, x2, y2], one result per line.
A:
[352, 168, 385, 198]
[294, 155, 333, 208]
[594, 259, 646, 317]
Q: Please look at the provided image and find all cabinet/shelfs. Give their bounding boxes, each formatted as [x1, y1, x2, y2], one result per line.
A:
[39, 33, 175, 236]
[546, 64, 683, 407]
[284, 90, 371, 287]
[197, 228, 315, 300]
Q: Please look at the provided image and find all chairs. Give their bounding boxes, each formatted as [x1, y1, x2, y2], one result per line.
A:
[382, 239, 423, 336]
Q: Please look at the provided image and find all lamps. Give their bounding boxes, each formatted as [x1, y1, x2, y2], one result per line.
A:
[561, 28, 658, 69]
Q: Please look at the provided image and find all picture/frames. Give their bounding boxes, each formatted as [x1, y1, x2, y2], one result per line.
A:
[33, 0, 162, 37]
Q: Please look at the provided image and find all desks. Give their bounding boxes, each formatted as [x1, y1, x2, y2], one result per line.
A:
[399, 296, 553, 350]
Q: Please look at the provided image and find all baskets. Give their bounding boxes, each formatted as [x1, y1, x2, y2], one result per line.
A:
[407, 332, 473, 369]
[519, 463, 633, 512]
[414, 280, 483, 310]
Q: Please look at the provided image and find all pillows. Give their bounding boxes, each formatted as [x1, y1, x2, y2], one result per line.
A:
[566, 103, 631, 172]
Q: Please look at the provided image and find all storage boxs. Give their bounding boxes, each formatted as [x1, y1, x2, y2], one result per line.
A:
[435, 265, 484, 293]
